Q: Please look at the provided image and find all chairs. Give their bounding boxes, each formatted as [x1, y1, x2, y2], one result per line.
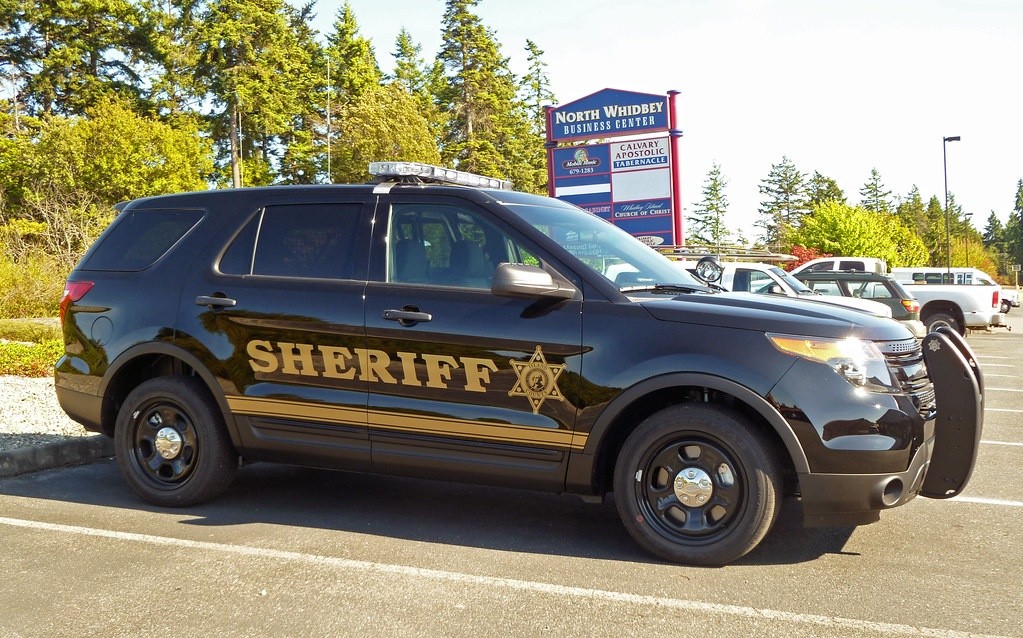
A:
[445, 239, 493, 289]
[396, 239, 438, 286]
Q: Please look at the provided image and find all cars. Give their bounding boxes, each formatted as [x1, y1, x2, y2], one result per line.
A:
[675, 255, 1022, 344]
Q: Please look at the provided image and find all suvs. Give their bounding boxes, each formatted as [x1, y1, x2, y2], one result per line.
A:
[50, 163, 985, 568]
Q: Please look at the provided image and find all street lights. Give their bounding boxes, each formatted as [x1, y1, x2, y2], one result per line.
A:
[941, 136, 961, 284]
[964, 211, 975, 267]
